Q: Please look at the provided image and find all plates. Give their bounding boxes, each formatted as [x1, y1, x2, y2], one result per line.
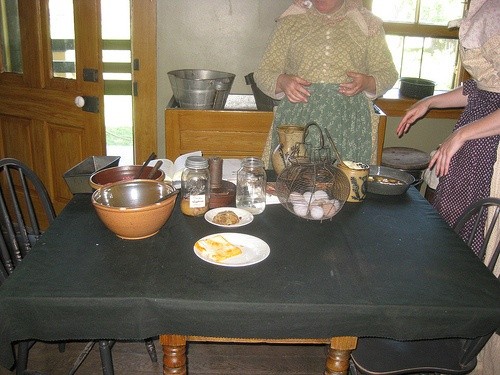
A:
[205, 207, 254, 228]
[193, 232, 272, 268]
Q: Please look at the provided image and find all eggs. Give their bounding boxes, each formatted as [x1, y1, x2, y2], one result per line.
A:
[288, 190, 340, 219]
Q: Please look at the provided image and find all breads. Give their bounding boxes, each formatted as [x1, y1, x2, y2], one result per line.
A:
[194, 234, 241, 262]
[213, 210, 238, 225]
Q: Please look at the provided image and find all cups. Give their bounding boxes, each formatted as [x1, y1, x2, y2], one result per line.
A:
[334, 161, 369, 202]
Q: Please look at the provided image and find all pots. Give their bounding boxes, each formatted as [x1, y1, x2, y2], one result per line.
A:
[366, 164, 423, 195]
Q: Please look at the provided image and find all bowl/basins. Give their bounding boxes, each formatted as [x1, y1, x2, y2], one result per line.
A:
[92, 179, 177, 240]
[88, 166, 166, 193]
[400, 76, 437, 100]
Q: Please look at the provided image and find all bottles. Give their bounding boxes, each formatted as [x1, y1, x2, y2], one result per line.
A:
[271, 124, 311, 178]
[205, 157, 236, 208]
[236, 157, 267, 215]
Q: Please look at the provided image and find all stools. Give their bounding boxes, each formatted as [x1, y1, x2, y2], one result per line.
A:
[380, 146, 430, 191]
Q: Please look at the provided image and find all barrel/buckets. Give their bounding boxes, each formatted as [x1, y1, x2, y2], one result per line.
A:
[168, 69, 236, 110]
[244, 74, 279, 112]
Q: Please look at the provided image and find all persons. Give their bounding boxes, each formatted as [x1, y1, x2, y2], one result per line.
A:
[254, 0, 399, 171]
[396, 0, 500, 278]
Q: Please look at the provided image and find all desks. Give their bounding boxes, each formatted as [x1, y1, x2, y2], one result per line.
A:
[0, 184, 500, 375]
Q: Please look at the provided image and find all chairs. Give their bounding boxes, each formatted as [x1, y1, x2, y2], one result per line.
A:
[348, 199, 500, 375]
[0, 157, 157, 375]
